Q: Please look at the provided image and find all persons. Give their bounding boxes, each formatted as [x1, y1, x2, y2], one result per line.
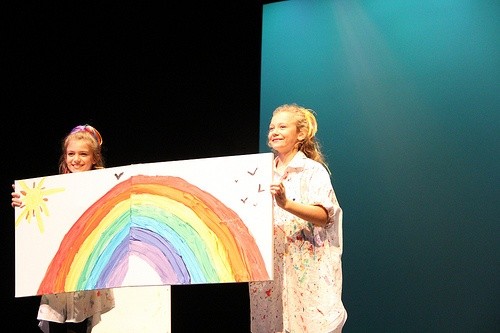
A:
[10, 123, 116, 333]
[248, 103, 348, 333]
